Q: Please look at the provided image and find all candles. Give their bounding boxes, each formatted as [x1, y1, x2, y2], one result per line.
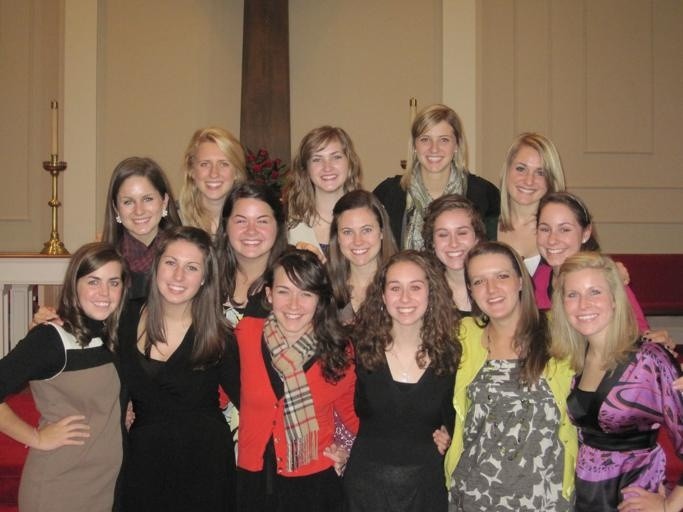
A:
[408, 97, 418, 122]
[49, 97, 60, 156]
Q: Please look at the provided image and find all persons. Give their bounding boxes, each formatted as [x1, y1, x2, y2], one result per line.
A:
[28, 227, 239, 512]
[442, 240, 682, 512]
[545, 254, 681, 512]
[339, 252, 456, 510]
[496, 131, 630, 286]
[282, 126, 363, 263]
[521, 193, 679, 361]
[328, 189, 399, 325]
[101, 156, 182, 302]
[0, 242, 135, 512]
[370, 104, 501, 253]
[420, 193, 488, 316]
[215, 184, 297, 468]
[218, 250, 450, 512]
[175, 126, 247, 237]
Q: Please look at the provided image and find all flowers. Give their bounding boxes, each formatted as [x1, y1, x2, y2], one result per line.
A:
[240, 145, 296, 191]
